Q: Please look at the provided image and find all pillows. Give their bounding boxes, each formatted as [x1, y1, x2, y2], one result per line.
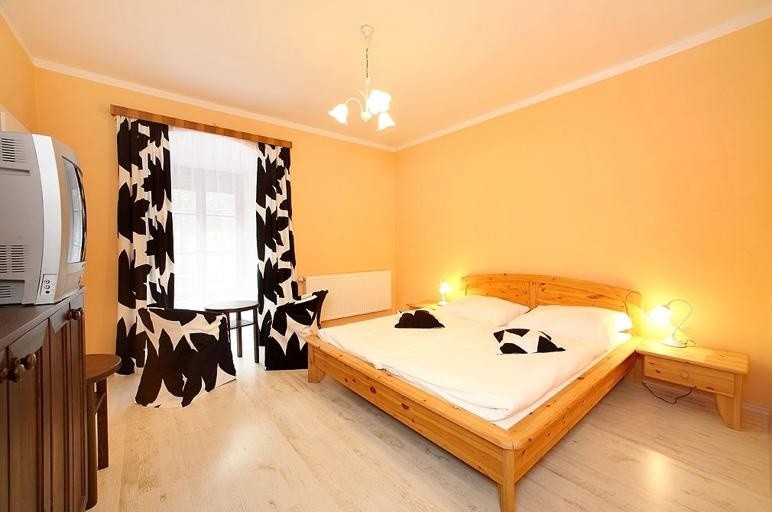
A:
[505, 304, 633, 351]
[436, 292, 531, 327]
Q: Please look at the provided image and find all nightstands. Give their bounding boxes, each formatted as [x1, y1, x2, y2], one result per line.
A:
[635, 338, 750, 432]
[404, 299, 453, 308]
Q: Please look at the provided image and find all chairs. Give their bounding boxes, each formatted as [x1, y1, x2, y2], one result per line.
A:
[259, 288, 328, 371]
[133, 305, 237, 408]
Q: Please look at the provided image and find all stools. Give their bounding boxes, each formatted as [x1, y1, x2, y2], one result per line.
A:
[86, 351, 122, 507]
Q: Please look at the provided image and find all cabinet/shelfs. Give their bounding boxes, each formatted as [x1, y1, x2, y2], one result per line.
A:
[0, 283, 86, 512]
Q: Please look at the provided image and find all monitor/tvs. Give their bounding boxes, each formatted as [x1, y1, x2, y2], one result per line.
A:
[0, 131, 88, 305]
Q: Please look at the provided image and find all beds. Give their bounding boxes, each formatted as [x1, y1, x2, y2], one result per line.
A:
[304, 273, 642, 512]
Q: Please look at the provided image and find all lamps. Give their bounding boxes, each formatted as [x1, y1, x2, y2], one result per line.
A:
[438, 280, 455, 307]
[328, 23, 397, 132]
[642, 297, 699, 350]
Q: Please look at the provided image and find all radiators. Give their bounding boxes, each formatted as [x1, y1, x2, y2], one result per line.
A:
[304, 271, 393, 322]
